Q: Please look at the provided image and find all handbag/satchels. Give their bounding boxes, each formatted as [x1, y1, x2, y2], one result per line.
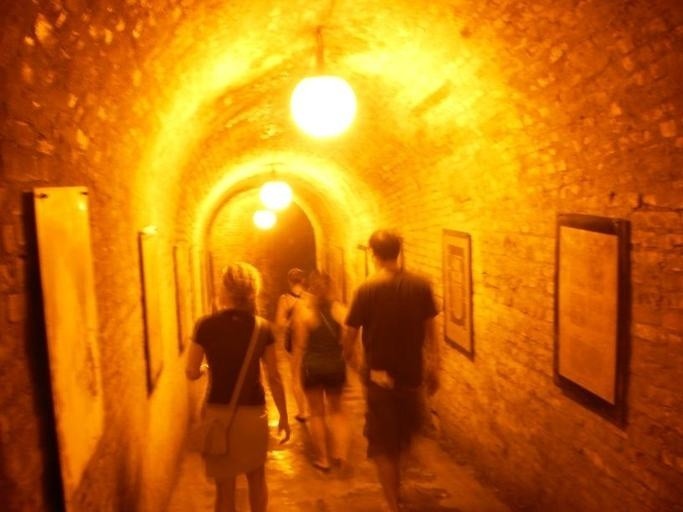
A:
[185, 415, 230, 459]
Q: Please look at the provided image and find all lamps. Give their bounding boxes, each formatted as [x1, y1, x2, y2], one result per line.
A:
[256, 162, 293, 215]
[285, 25, 358, 143]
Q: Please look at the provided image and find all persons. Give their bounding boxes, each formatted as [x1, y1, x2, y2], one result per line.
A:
[184, 260, 290, 512]
[277, 267, 312, 422]
[340, 229, 441, 512]
[291, 270, 349, 472]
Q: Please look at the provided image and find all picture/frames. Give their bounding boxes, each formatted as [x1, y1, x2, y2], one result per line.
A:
[172, 243, 214, 355]
[137, 231, 165, 397]
[438, 228, 477, 359]
[549, 211, 632, 414]
[24, 181, 113, 512]
[319, 237, 402, 310]
